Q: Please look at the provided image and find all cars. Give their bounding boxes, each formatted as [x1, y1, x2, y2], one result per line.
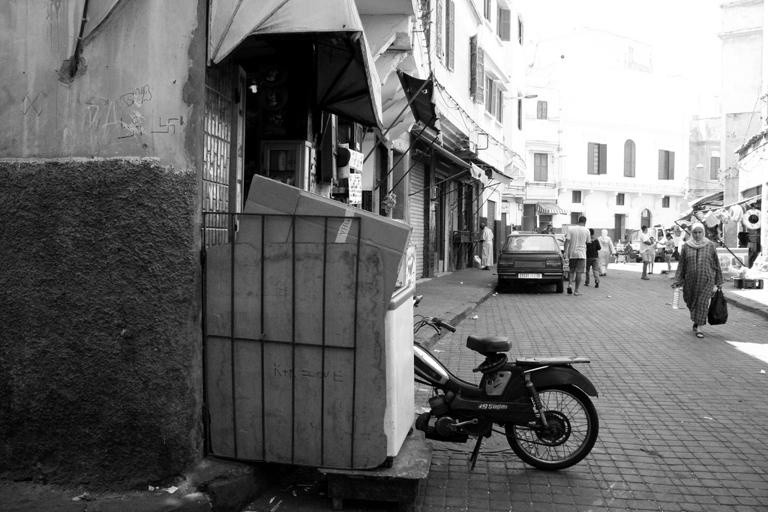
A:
[497, 234, 564, 292]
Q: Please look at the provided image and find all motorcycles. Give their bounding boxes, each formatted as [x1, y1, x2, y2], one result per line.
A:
[414, 294, 600, 474]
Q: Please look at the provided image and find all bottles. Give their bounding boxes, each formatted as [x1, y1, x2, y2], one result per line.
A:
[673, 287, 683, 309]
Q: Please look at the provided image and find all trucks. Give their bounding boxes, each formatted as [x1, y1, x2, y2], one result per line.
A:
[614, 227, 666, 262]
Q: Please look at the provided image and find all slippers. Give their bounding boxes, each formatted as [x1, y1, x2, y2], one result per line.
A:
[567, 288, 585, 296]
[694, 323, 704, 338]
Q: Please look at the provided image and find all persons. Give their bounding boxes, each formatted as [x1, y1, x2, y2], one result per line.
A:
[655, 232, 674, 270]
[669, 223, 723, 339]
[647, 237, 655, 274]
[584, 227, 601, 288]
[597, 229, 616, 276]
[478, 223, 494, 270]
[639, 225, 651, 280]
[560, 216, 587, 297]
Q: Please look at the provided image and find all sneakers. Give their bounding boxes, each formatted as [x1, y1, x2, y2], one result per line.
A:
[641, 273, 653, 280]
[584, 271, 606, 288]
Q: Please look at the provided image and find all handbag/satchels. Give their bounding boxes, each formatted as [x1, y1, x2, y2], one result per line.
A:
[708, 289, 728, 325]
